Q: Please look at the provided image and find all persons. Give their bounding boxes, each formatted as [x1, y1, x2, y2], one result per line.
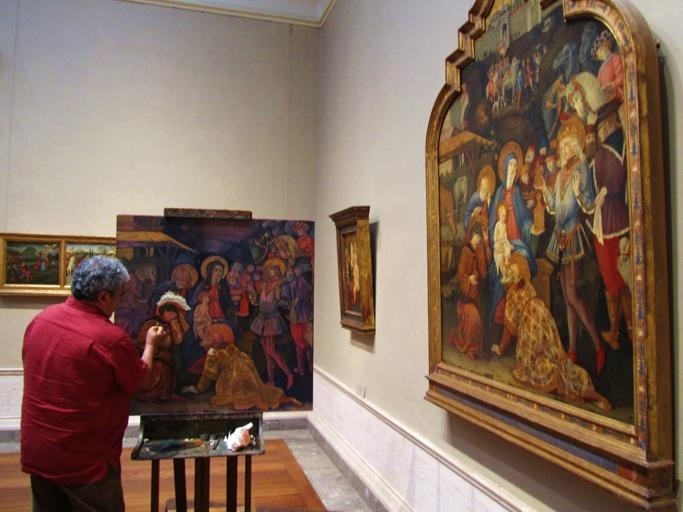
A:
[116, 220, 314, 412]
[20, 254, 167, 512]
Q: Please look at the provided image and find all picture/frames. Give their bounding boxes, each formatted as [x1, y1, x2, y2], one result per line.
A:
[423, 0, 677, 512]
[0, 232, 117, 298]
[328, 205, 375, 335]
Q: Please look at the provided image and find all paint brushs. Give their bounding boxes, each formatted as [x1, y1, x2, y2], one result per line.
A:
[213, 439, 221, 450]
[155, 321, 158, 344]
